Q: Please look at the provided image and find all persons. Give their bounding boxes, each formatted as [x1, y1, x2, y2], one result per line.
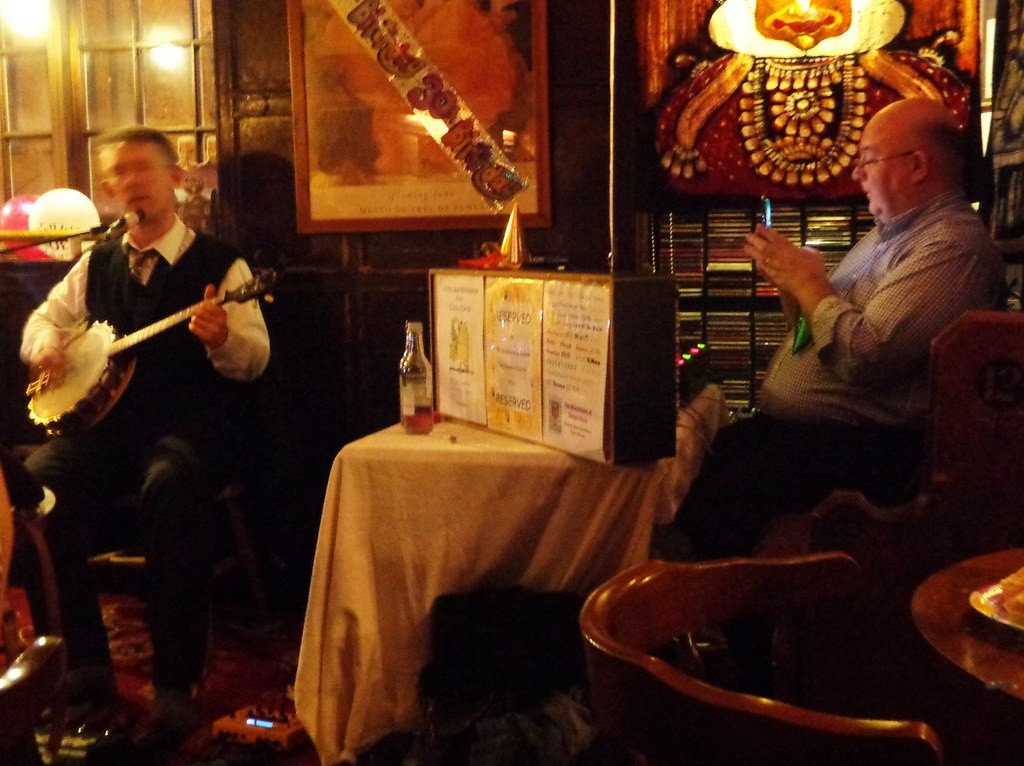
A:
[19, 124, 271, 750]
[675, 98, 1009, 563]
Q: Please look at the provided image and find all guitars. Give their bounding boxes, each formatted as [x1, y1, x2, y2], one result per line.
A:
[26, 267, 278, 440]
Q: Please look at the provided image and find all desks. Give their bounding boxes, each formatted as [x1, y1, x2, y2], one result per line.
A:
[910, 546, 1024, 700]
[295, 380, 725, 766]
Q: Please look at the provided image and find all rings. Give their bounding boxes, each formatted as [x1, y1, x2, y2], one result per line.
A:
[39, 364, 43, 370]
[761, 258, 770, 265]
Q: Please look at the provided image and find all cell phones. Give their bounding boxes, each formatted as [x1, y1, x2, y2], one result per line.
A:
[761, 194, 771, 228]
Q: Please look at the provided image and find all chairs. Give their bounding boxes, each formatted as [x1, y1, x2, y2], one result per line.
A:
[80, 465, 283, 654]
[578, 551, 945, 766]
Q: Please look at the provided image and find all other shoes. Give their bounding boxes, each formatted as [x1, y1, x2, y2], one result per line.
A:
[73, 666, 118, 699]
[130, 678, 204, 752]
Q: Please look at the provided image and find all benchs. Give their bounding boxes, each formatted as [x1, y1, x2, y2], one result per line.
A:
[752, 310, 1024, 547]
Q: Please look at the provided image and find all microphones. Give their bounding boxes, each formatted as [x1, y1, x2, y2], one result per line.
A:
[103, 209, 145, 242]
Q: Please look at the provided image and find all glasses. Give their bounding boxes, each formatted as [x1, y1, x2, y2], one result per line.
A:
[853, 150, 915, 173]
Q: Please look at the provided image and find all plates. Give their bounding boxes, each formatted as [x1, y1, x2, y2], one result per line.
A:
[970, 582, 1024, 633]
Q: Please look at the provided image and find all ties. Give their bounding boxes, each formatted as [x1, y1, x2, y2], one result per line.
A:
[125, 242, 158, 281]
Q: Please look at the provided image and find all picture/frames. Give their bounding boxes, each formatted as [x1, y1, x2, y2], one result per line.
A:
[289, 0, 553, 235]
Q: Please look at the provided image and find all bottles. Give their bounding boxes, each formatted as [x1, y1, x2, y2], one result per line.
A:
[399, 323, 434, 435]
